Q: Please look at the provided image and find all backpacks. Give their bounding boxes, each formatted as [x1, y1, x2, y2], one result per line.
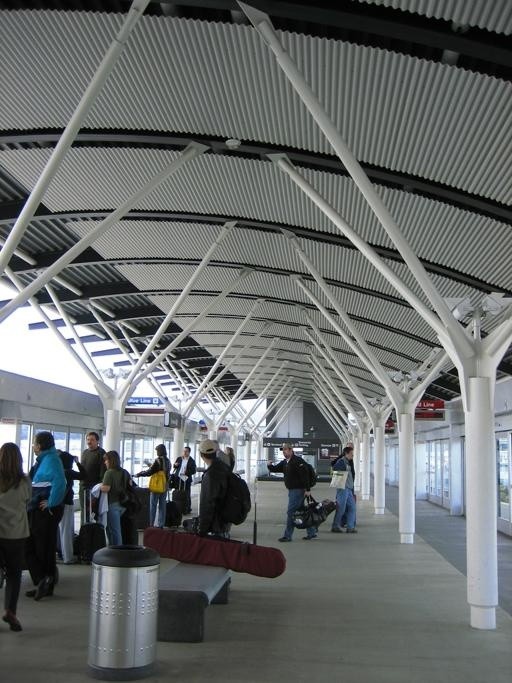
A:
[208, 461, 250, 525]
[307, 464, 316, 488]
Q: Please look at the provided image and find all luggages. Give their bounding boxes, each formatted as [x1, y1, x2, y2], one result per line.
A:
[121, 517, 138, 545]
[130, 475, 149, 528]
[79, 490, 106, 565]
[172, 474, 189, 515]
[315, 499, 336, 531]
[165, 490, 182, 527]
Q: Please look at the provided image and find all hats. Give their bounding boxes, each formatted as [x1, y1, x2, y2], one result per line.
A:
[279, 443, 292, 450]
[199, 438, 216, 454]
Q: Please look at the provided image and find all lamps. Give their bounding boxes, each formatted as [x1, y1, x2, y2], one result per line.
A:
[387, 368, 426, 383]
[443, 291, 512, 321]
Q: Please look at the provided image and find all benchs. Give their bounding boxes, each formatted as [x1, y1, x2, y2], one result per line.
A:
[154, 560, 232, 642]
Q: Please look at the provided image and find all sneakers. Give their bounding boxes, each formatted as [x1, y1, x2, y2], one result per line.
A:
[3, 556, 79, 632]
[278, 522, 358, 542]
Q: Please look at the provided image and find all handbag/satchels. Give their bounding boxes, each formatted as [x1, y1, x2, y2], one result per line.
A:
[169, 468, 179, 489]
[330, 470, 349, 490]
[292, 495, 327, 529]
[149, 458, 166, 493]
[120, 470, 141, 512]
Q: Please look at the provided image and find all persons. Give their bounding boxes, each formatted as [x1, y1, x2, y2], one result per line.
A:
[331, 443, 355, 526]
[0, 441, 33, 631]
[330, 447, 359, 533]
[56, 430, 236, 605]
[267, 442, 319, 542]
[26, 431, 68, 600]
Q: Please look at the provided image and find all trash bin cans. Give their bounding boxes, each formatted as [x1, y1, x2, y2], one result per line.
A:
[85, 544, 160, 682]
